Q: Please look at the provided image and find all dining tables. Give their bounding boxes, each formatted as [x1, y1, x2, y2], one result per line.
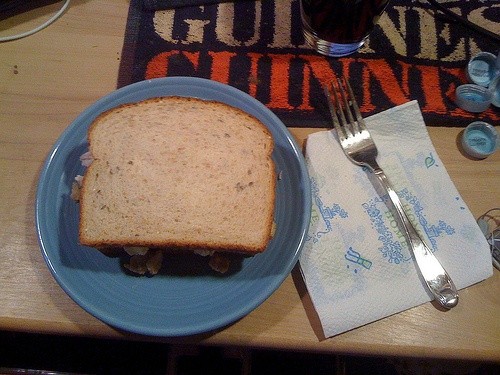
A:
[0, 0, 500, 364]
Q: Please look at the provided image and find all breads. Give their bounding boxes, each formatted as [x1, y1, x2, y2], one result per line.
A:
[77, 96, 276, 255]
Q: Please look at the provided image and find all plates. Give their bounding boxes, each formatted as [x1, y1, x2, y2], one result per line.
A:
[34, 76, 313, 337]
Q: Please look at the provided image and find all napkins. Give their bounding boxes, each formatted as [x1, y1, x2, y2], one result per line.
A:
[298, 100, 493, 338]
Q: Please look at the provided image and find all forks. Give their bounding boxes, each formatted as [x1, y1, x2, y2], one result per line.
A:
[324, 75, 459, 308]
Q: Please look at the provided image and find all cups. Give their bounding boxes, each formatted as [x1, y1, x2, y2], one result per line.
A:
[299, 0, 392, 57]
